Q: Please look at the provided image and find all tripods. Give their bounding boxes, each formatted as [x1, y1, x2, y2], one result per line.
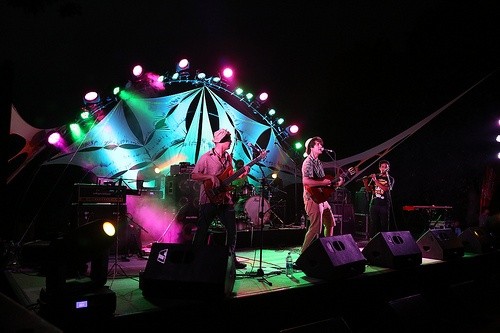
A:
[275, 141, 305, 227]
[98, 176, 142, 290]
[233, 158, 283, 285]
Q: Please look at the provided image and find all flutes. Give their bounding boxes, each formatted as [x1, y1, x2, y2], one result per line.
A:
[356, 171, 386, 182]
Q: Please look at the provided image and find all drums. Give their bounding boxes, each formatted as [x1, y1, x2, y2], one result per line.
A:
[229, 184, 240, 204]
[241, 184, 256, 198]
[235, 196, 272, 226]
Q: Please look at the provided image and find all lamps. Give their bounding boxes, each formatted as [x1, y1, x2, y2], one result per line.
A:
[274, 116, 285, 127]
[458, 227, 480, 250]
[83, 90, 102, 107]
[133, 65, 147, 78]
[256, 91, 268, 105]
[18, 221, 117, 328]
[175, 57, 191, 81]
[286, 123, 299, 137]
[267, 107, 277, 117]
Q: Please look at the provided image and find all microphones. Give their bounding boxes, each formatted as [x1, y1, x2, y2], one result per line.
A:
[248, 142, 261, 153]
[382, 168, 388, 173]
[320, 148, 334, 153]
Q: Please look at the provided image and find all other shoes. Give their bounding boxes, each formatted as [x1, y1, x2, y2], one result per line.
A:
[234, 259, 247, 269]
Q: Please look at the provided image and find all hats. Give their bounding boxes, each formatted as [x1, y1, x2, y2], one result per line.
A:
[304, 137, 323, 153]
[211, 129, 230, 143]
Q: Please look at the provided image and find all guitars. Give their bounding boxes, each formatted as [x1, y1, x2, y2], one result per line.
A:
[306, 166, 359, 205]
[204, 149, 270, 208]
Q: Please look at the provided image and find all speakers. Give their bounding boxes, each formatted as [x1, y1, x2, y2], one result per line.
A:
[141, 242, 235, 304]
[417, 228, 465, 260]
[362, 231, 422, 269]
[295, 233, 366, 279]
[331, 204, 355, 235]
[165, 176, 202, 216]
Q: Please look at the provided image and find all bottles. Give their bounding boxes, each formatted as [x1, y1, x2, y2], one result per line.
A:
[285, 253, 292, 276]
[301, 215, 305, 229]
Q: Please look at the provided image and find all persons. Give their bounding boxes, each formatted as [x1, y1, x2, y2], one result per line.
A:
[300, 135, 342, 253]
[230, 160, 248, 191]
[362, 159, 395, 241]
[191, 129, 251, 269]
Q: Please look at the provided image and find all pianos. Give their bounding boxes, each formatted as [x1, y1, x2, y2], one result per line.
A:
[403, 205, 453, 228]
